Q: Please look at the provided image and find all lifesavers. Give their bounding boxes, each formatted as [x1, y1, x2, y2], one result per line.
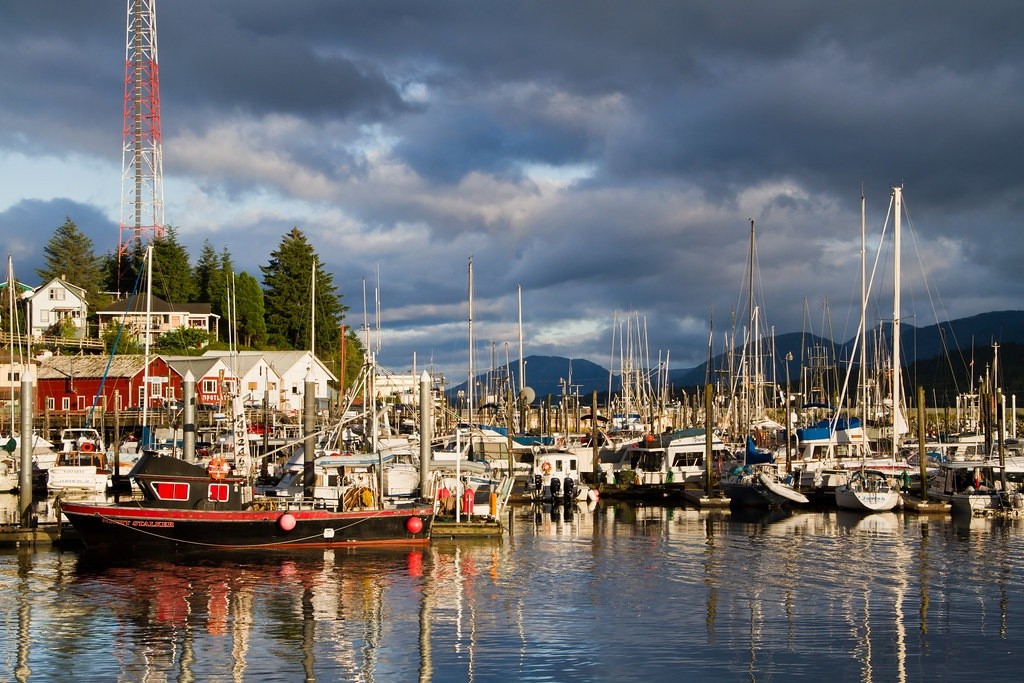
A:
[81, 443, 93, 452]
[208, 459, 230, 479]
[542, 463, 552, 471]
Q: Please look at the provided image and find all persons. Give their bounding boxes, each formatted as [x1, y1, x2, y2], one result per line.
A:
[597, 458, 603, 484]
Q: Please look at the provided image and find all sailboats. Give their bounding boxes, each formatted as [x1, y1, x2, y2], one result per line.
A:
[0, 183, 1024, 558]
